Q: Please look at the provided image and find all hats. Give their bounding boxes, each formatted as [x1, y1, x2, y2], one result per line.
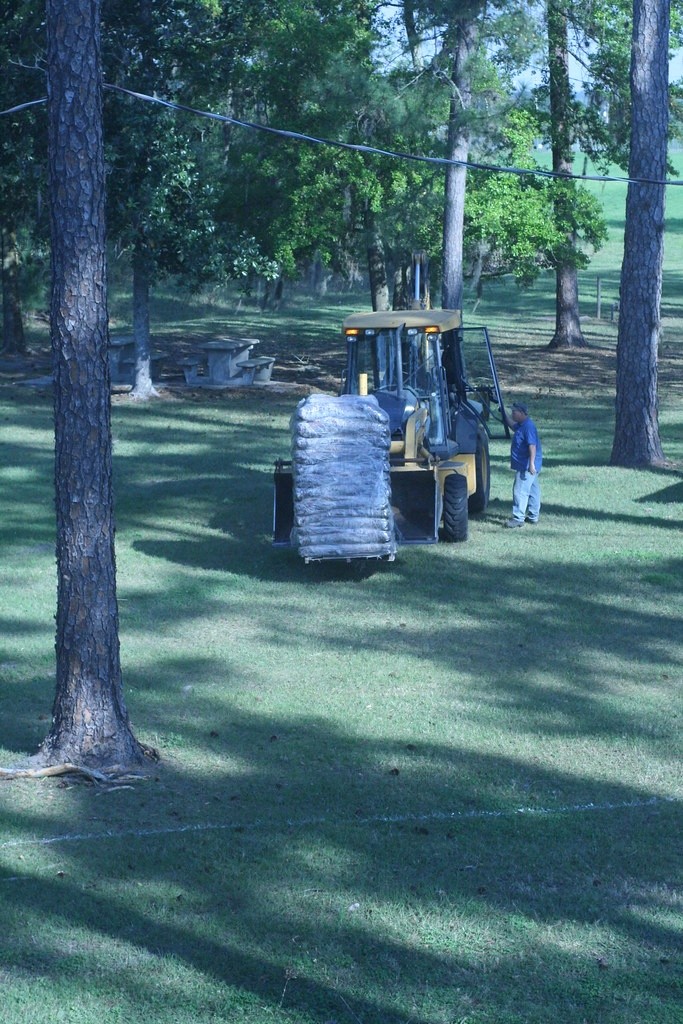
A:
[506, 403, 528, 413]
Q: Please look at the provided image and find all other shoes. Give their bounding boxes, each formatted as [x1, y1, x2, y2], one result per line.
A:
[505, 519, 525, 527]
[525, 517, 539, 524]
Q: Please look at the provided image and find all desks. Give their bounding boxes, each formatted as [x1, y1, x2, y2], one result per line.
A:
[198, 337, 261, 385]
[110, 332, 153, 384]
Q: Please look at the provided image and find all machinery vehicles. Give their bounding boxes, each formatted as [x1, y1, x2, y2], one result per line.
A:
[270, 310, 512, 547]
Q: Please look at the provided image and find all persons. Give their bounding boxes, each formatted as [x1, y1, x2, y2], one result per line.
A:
[498, 403, 542, 528]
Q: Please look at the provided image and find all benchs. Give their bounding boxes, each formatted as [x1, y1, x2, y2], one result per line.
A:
[237, 356, 277, 384]
[179, 354, 209, 387]
[123, 351, 173, 382]
